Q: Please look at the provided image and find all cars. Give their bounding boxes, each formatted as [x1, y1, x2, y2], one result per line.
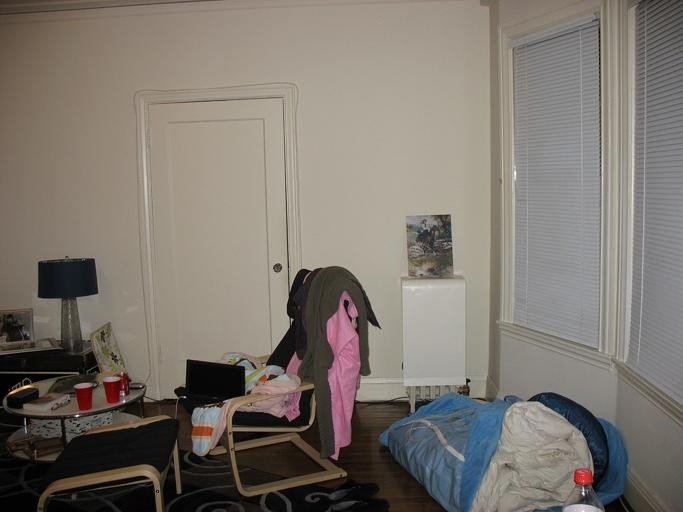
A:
[73, 383, 93, 411]
[102, 377, 121, 403]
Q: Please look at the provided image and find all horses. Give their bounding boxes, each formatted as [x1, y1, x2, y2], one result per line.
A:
[129, 382, 145, 389]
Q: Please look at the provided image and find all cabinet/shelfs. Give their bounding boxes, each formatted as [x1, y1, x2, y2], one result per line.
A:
[561, 468, 605, 512]
[117, 372, 129, 396]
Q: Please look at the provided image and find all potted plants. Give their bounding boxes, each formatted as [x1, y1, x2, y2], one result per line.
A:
[0, 305, 36, 351]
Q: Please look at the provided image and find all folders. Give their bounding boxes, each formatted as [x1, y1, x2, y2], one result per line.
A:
[402, 279, 471, 414]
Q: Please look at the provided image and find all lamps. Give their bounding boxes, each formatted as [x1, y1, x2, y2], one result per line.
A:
[175, 268, 348, 496]
[34, 415, 178, 511]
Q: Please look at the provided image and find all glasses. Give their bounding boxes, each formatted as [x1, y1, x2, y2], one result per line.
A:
[37, 254, 99, 353]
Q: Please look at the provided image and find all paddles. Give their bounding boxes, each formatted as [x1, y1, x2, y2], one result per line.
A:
[1, 440, 390, 512]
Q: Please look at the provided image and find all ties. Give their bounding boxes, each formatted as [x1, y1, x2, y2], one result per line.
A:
[186, 359, 246, 403]
